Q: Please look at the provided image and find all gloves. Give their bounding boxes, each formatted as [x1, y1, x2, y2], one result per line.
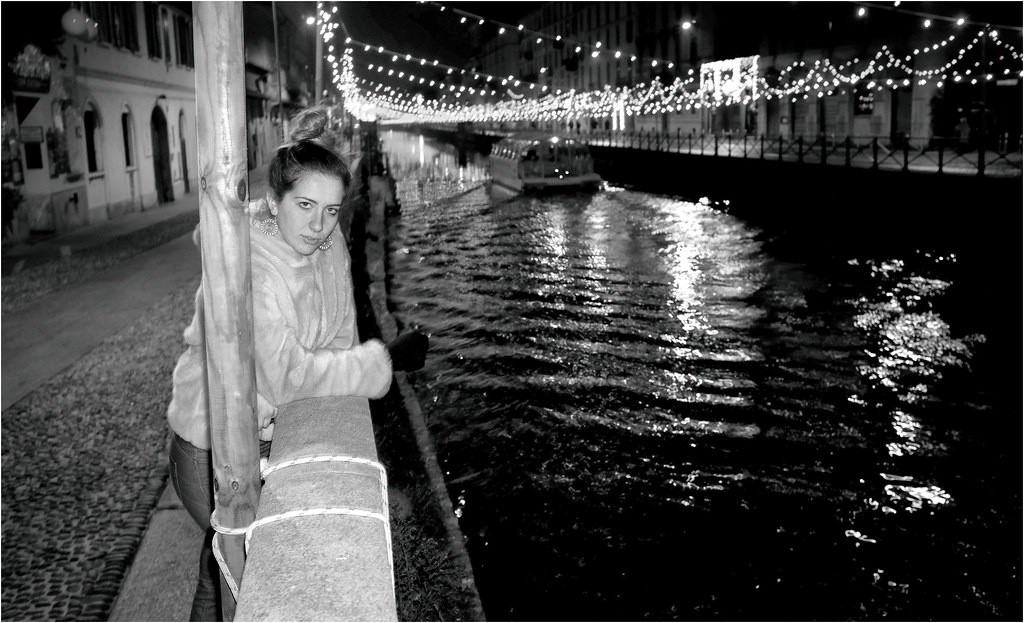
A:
[385, 332, 428, 374]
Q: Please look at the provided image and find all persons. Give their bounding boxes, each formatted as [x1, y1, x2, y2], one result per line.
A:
[167, 108, 431, 623]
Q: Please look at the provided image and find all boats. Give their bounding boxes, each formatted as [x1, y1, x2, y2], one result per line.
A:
[490, 129, 601, 198]
